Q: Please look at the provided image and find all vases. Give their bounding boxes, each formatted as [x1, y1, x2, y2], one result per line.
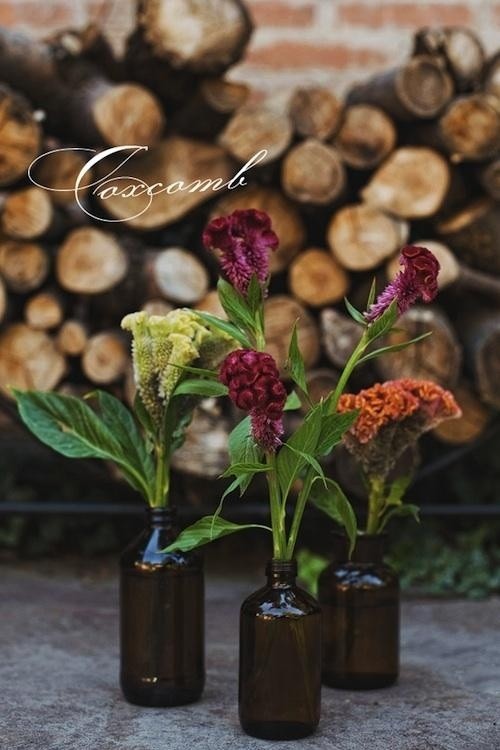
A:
[118, 507, 403, 740]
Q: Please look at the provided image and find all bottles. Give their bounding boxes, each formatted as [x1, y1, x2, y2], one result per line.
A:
[238, 560, 325, 739]
[117, 506, 206, 707]
[318, 531, 402, 692]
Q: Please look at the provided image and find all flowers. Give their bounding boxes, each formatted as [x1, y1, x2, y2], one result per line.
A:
[5, 206, 463, 728]
